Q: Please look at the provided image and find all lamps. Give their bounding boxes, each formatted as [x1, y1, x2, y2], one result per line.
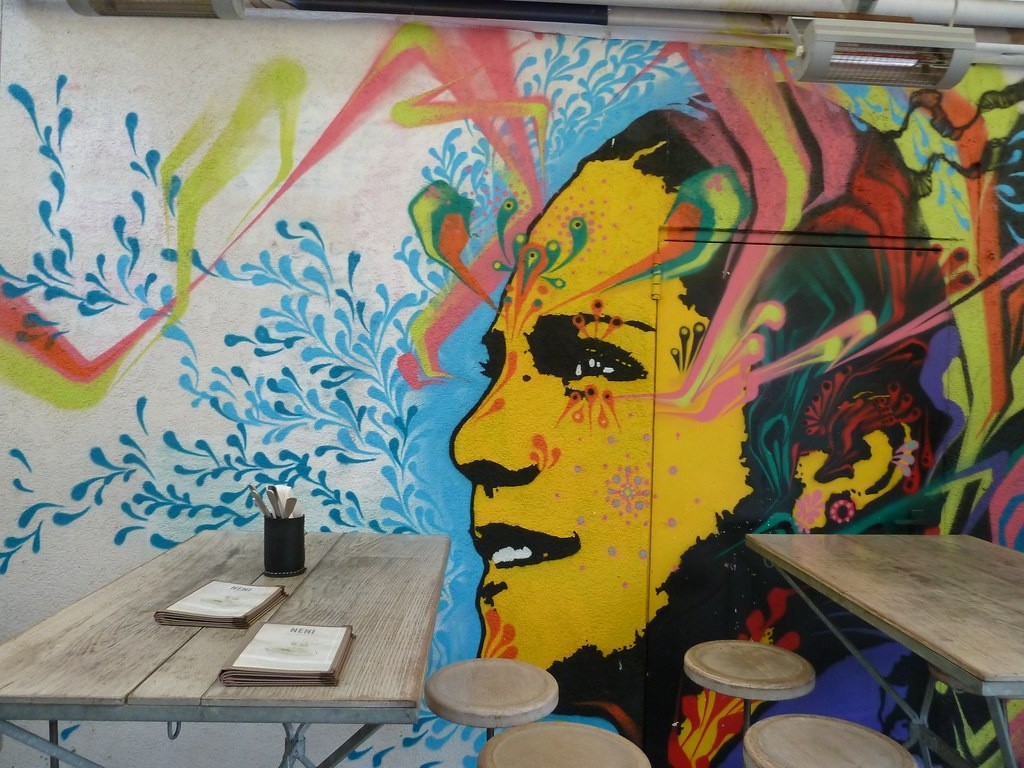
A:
[786, 16, 976, 91]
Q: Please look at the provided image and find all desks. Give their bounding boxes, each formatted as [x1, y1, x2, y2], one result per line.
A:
[744, 533, 1024, 768]
[0, 530, 453, 768]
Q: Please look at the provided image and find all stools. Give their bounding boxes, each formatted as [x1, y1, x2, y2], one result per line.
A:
[682, 639, 816, 739]
[742, 713, 922, 768]
[424, 659, 559, 742]
[479, 721, 653, 768]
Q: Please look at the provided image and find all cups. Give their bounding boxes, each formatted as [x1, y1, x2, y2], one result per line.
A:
[264, 513, 306, 577]
